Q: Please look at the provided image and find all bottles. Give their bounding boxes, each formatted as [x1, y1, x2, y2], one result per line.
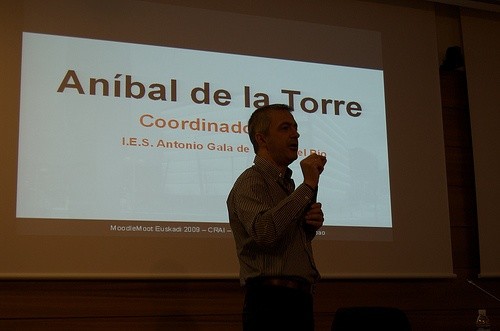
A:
[476, 309, 490, 331]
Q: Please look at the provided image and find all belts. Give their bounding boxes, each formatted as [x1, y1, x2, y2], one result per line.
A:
[262, 278, 316, 295]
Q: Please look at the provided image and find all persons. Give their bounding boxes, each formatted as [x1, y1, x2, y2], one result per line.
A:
[227, 103, 327, 331]
